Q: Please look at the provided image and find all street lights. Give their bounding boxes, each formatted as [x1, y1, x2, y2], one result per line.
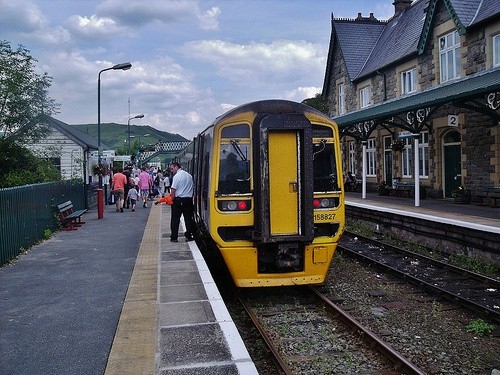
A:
[96, 62, 132, 222]
[127, 113, 151, 170]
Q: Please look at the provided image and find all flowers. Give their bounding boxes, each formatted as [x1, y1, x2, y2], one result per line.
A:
[92, 163, 110, 177]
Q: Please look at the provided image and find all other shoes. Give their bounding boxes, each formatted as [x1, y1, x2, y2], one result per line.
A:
[117, 209, 119, 212]
[132, 208, 135, 211]
[120, 208, 124, 213]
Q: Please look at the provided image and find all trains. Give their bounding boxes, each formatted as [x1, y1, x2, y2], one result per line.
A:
[169, 99, 347, 287]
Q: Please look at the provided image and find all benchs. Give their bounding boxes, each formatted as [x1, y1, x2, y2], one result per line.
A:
[348, 181, 363, 192]
[57, 201, 87, 230]
[477, 187, 500, 207]
[394, 181, 417, 197]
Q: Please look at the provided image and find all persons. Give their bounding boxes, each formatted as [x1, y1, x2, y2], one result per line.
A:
[343, 172, 360, 191]
[111, 157, 173, 212]
[169, 162, 196, 242]
[392, 177, 403, 197]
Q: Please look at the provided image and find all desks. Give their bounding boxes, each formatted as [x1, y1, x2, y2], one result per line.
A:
[454, 189, 471, 204]
[378, 186, 389, 196]
